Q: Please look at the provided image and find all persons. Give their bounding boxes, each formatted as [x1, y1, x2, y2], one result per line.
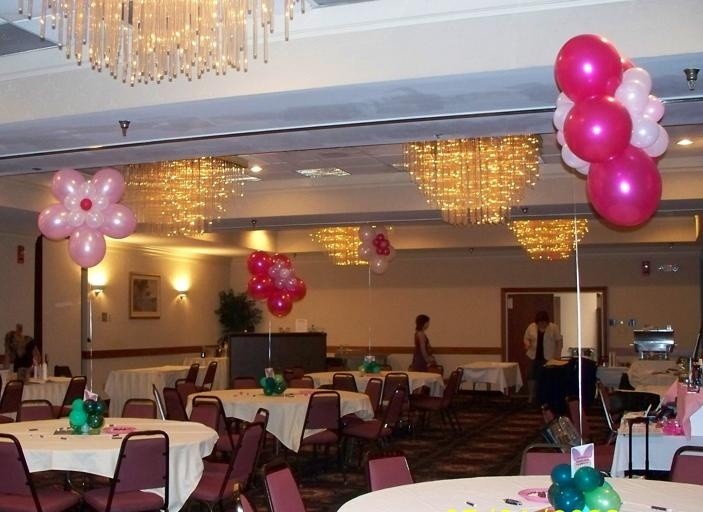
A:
[3, 331, 41, 372]
[407, 314, 436, 372]
[523, 310, 563, 403]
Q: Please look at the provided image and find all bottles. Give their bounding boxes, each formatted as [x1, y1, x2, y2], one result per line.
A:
[33, 360, 47, 381]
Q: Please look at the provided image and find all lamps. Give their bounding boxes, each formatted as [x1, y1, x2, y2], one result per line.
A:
[17, 0, 305, 88]
[117, 156, 249, 237]
[309, 226, 393, 266]
[402, 134, 543, 226]
[170, 269, 191, 300]
[87, 259, 110, 297]
[506, 217, 589, 261]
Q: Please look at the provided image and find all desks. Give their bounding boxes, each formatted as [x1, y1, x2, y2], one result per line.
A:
[456, 361, 524, 403]
[628, 359, 678, 388]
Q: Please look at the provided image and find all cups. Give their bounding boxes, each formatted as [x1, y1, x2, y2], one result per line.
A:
[17, 367, 29, 382]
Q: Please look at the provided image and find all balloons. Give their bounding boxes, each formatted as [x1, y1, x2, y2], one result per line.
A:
[358, 361, 380, 377]
[547, 463, 623, 512]
[247, 250, 307, 318]
[552, 34, 669, 228]
[69, 398, 107, 434]
[358, 223, 396, 276]
[37, 168, 138, 269]
[259, 374, 287, 396]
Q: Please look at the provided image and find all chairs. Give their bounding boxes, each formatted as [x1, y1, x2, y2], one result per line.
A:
[668, 446, 703, 486]
[520, 380, 620, 475]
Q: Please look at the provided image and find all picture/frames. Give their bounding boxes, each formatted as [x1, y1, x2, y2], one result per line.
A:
[129, 271, 161, 319]
